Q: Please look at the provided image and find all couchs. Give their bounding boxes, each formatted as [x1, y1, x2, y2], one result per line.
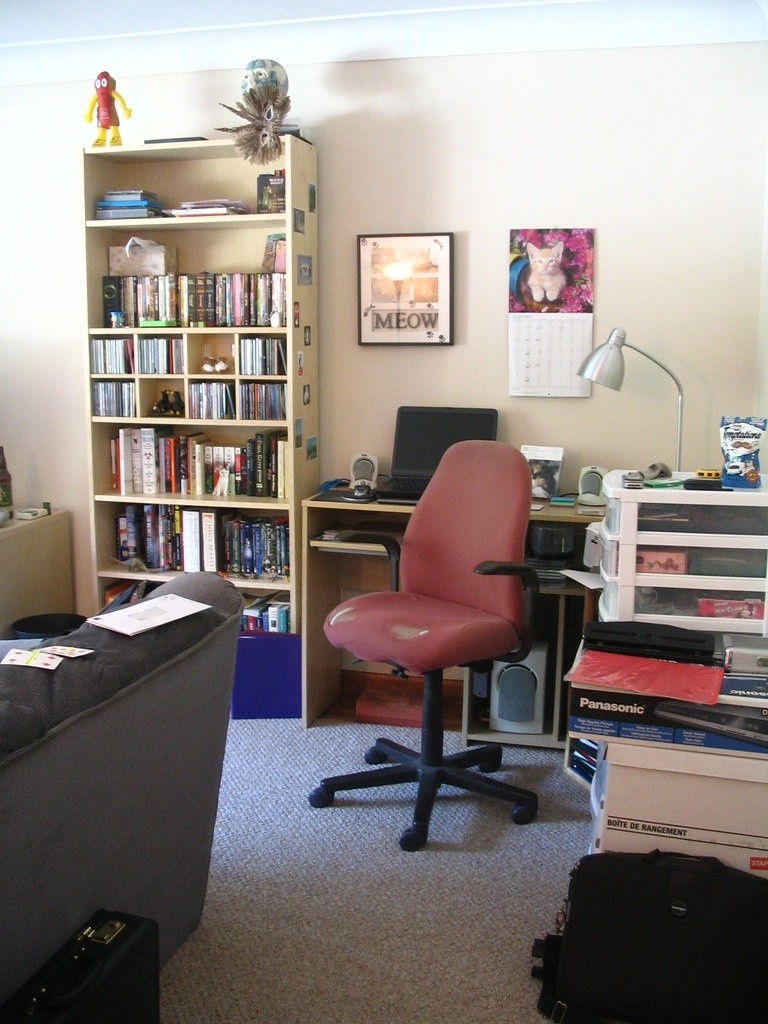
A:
[1, 573, 244, 1004]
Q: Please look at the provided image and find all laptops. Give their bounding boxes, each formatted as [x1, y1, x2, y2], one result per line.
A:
[373, 406, 498, 499]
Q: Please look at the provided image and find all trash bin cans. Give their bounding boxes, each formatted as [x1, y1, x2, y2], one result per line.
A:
[13, 614, 87, 639]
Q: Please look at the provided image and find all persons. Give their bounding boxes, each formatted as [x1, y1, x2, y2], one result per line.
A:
[304, 386, 309, 404]
[299, 358, 303, 376]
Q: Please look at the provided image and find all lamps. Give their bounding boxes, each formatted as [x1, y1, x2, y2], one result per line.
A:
[576, 327, 684, 472]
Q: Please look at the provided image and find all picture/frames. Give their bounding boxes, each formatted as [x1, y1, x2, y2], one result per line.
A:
[357, 232, 454, 346]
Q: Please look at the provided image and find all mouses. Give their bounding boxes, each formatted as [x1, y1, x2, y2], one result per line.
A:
[354, 485, 369, 497]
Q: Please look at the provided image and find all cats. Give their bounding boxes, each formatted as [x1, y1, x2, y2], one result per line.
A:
[526, 241, 567, 303]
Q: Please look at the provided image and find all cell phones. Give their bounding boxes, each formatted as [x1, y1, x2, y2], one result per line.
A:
[621, 475, 643, 489]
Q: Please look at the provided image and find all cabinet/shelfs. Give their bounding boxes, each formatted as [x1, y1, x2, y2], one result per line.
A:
[599, 469, 768, 657]
[79, 133, 342, 730]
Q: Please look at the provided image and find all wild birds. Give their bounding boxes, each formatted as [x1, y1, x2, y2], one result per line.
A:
[152, 389, 185, 414]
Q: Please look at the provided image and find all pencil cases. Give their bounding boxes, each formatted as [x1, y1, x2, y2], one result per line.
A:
[684, 479, 722, 490]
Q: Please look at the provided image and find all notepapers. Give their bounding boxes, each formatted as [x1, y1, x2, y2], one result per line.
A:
[550, 496, 576, 507]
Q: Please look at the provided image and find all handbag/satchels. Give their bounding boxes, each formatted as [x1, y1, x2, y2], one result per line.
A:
[532, 850, 768, 1024]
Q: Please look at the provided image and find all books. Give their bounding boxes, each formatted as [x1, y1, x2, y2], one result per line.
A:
[109, 427, 288, 499]
[115, 504, 290, 584]
[90, 335, 287, 376]
[96, 187, 252, 220]
[103, 272, 287, 328]
[91, 380, 287, 421]
[106, 583, 291, 633]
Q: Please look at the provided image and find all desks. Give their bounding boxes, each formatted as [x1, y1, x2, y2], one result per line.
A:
[301, 486, 608, 751]
[0, 507, 74, 640]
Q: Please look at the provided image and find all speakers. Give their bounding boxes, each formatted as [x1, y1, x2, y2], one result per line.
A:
[488, 641, 549, 734]
[349, 454, 379, 490]
[578, 466, 608, 505]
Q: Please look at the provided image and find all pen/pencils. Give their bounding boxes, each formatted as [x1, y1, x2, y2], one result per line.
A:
[722, 489, 733, 491]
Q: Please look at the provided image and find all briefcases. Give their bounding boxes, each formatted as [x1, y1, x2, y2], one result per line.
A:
[1, 908, 161, 1024]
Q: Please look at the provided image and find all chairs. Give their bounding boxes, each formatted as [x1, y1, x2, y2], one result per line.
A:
[307, 440, 540, 854]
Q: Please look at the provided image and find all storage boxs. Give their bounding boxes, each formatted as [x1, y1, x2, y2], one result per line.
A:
[356, 676, 463, 730]
[693, 592, 764, 619]
[636, 548, 688, 575]
[565, 633, 768, 878]
